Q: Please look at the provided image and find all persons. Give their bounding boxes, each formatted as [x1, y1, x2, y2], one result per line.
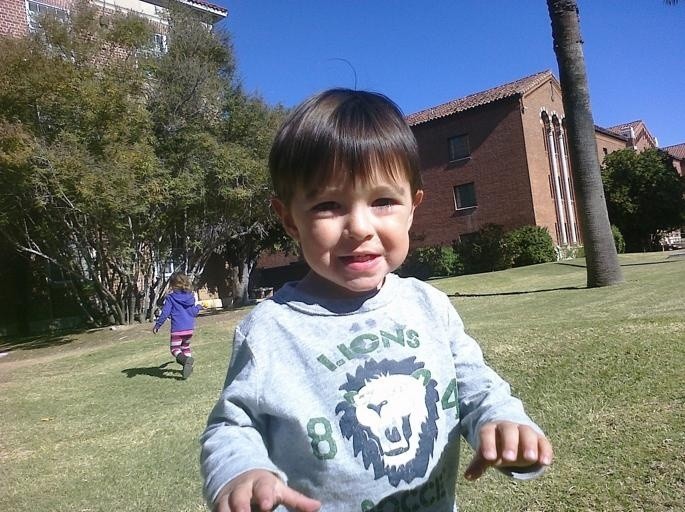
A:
[152, 273, 204, 380]
[198, 87, 552, 512]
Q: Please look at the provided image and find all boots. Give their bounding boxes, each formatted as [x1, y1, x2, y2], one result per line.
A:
[176, 352, 194, 379]
